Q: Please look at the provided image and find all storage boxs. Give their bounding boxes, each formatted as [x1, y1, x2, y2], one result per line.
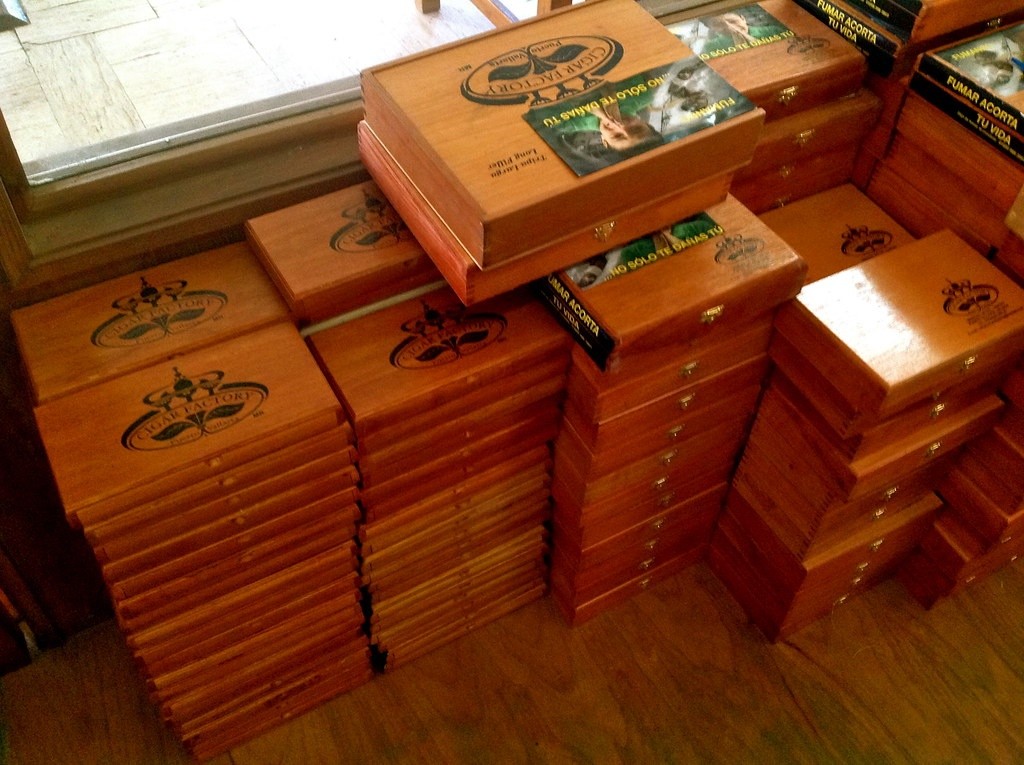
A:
[7, 0, 1024, 765]
